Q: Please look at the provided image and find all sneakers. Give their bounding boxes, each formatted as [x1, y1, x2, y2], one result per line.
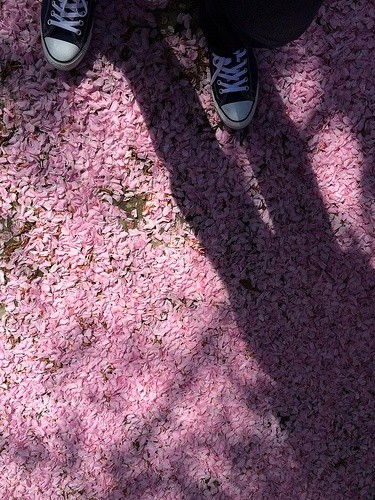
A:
[207, 46, 261, 131]
[39, 0, 95, 72]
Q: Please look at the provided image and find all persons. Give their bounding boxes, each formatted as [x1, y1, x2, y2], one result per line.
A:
[39, 1, 325, 130]
